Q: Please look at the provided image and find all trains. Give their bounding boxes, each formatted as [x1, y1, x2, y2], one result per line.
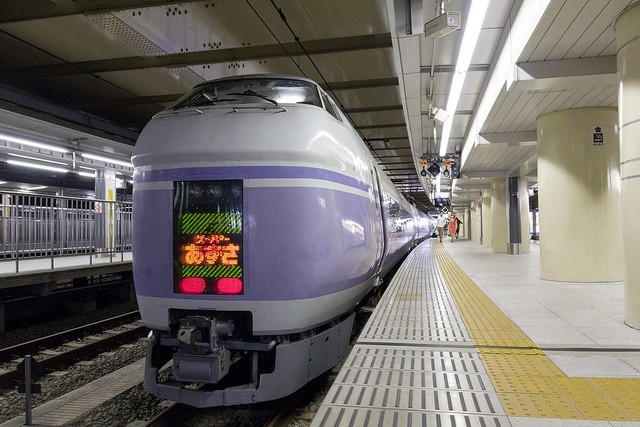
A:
[0, 144, 133, 266]
[130, 72, 439, 411]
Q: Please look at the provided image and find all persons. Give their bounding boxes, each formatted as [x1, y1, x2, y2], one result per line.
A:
[455, 217, 463, 240]
[448, 212, 458, 242]
[436, 211, 446, 244]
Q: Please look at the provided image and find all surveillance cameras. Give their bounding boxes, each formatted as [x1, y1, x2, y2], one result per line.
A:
[423, 11, 462, 40]
